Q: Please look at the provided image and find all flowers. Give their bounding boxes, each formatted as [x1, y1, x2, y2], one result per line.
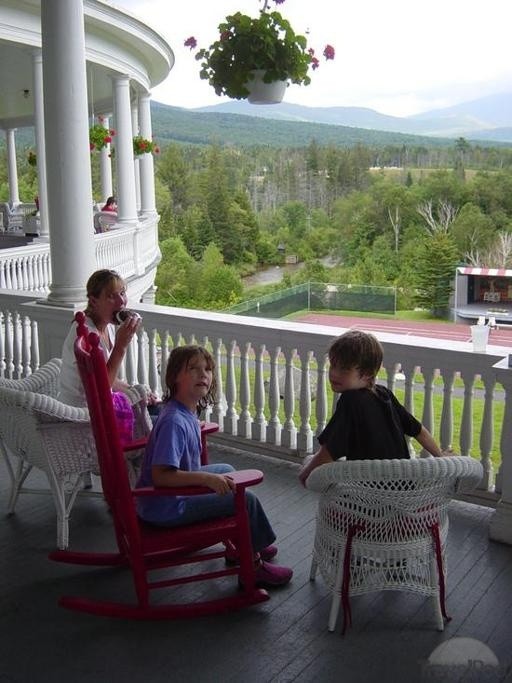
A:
[28, 150, 36, 167]
[185, 0, 337, 98]
[90, 115, 162, 159]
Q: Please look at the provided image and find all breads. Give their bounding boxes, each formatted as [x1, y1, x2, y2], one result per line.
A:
[112, 310, 142, 325]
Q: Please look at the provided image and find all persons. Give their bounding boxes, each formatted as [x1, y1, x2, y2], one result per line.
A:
[296, 330, 443, 586]
[56, 271, 158, 467]
[101, 196, 118, 212]
[129, 346, 294, 587]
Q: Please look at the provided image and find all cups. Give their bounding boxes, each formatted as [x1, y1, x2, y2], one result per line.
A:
[471, 325, 491, 353]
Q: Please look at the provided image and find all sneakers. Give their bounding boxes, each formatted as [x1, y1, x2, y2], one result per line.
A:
[225, 543, 293, 586]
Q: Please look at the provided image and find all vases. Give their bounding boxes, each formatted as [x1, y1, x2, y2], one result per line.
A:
[242, 68, 289, 103]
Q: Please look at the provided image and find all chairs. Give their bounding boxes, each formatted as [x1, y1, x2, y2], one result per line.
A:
[48, 310, 272, 620]
[2, 356, 156, 551]
[4, 201, 25, 232]
[304, 448, 484, 633]
[93, 210, 118, 233]
